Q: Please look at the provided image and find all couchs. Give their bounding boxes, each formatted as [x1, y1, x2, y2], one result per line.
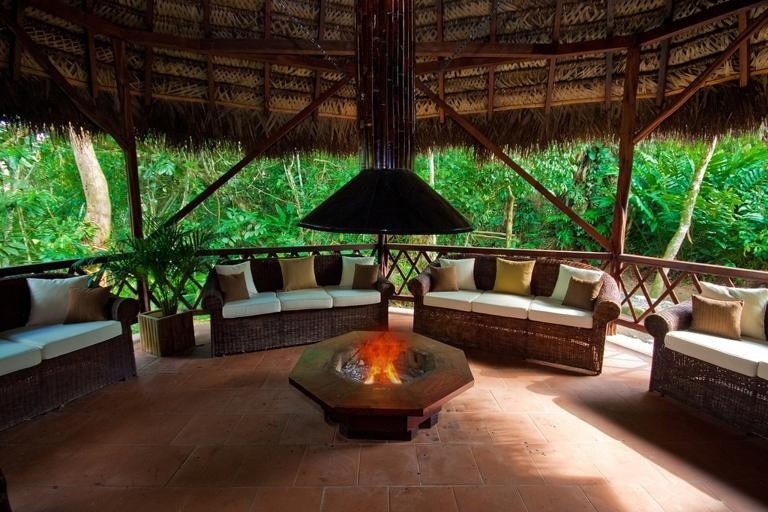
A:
[645, 280, 767, 441]
[408, 253, 621, 376]
[0, 273, 140, 433]
[201, 254, 395, 356]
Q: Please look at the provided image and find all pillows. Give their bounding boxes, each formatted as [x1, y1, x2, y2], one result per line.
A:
[352, 264, 380, 289]
[493, 257, 536, 296]
[26, 275, 88, 328]
[214, 261, 258, 297]
[429, 266, 458, 291]
[552, 264, 604, 311]
[218, 272, 249, 302]
[439, 258, 476, 290]
[277, 255, 318, 291]
[691, 282, 768, 341]
[340, 255, 376, 288]
[0, 273, 64, 330]
[63, 285, 112, 323]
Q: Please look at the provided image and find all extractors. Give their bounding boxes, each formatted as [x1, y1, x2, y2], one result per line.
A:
[296, 0, 479, 235]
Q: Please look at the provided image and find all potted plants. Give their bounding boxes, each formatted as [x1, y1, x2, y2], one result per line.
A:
[70, 222, 218, 358]
